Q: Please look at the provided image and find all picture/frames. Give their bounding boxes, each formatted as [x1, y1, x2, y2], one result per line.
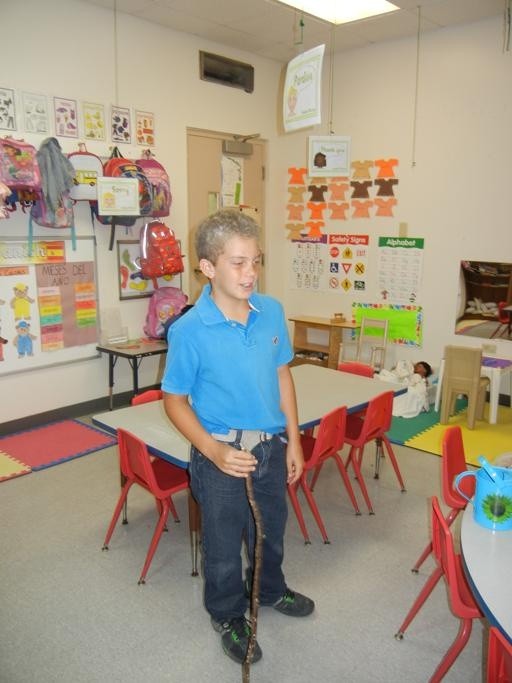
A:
[308, 135, 352, 178]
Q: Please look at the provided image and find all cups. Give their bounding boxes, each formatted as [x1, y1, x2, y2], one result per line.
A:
[456, 466, 512, 530]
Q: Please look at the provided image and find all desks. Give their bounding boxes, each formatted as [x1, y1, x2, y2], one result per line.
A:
[95, 336, 169, 410]
[91, 364, 407, 576]
[459, 465, 512, 646]
[288, 315, 361, 368]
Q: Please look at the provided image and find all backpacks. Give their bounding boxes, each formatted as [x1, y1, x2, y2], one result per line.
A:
[135, 149, 172, 217]
[143, 287, 188, 340]
[66, 143, 105, 200]
[0, 136, 43, 201]
[103, 147, 152, 219]
[30, 188, 75, 228]
[140, 220, 184, 280]
[89, 161, 137, 226]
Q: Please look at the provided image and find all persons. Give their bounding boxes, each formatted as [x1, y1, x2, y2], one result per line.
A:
[161, 208, 315, 663]
[413, 362, 431, 377]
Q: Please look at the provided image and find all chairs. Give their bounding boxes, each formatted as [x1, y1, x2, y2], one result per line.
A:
[394, 497, 484, 682]
[412, 427, 478, 574]
[102, 428, 190, 586]
[344, 390, 406, 516]
[338, 362, 374, 378]
[486, 625, 511, 682]
[287, 479, 311, 545]
[440, 345, 490, 429]
[130, 390, 165, 406]
[284, 407, 362, 546]
[337, 318, 388, 370]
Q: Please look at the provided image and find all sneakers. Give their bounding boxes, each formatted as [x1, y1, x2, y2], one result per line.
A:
[211, 615, 262, 663]
[244, 579, 314, 617]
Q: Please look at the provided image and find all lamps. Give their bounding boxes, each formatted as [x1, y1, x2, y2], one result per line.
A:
[271, 0, 402, 25]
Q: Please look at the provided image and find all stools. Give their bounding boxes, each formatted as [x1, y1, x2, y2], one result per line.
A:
[434, 356, 512, 424]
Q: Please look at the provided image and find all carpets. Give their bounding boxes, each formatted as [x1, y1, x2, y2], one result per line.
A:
[383, 397, 512, 466]
[1, 403, 132, 482]
